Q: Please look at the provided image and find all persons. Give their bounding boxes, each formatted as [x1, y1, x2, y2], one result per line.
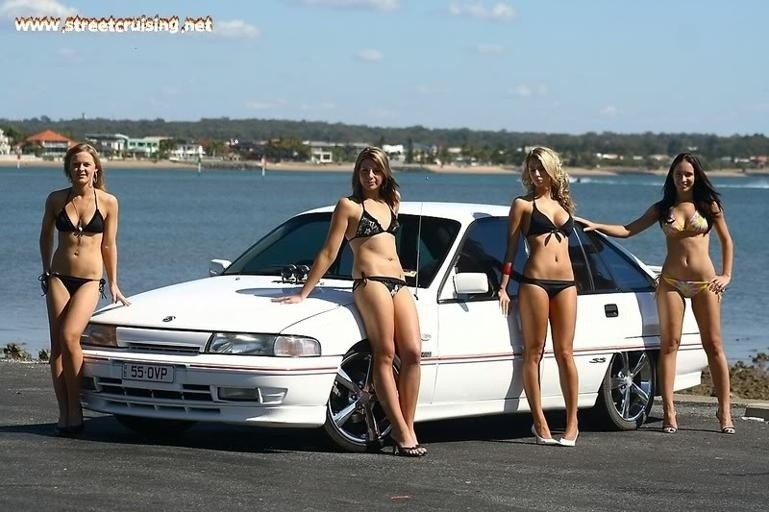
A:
[271, 147, 427, 458]
[572, 152, 734, 434]
[38, 143, 130, 435]
[498, 147, 579, 447]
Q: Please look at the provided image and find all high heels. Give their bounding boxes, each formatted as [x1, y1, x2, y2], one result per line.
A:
[663, 409, 678, 435]
[716, 406, 736, 434]
[558, 429, 581, 448]
[386, 433, 427, 456]
[531, 423, 557, 446]
[55, 413, 84, 433]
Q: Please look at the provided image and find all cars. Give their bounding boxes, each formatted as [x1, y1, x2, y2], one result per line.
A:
[78, 202, 711, 451]
